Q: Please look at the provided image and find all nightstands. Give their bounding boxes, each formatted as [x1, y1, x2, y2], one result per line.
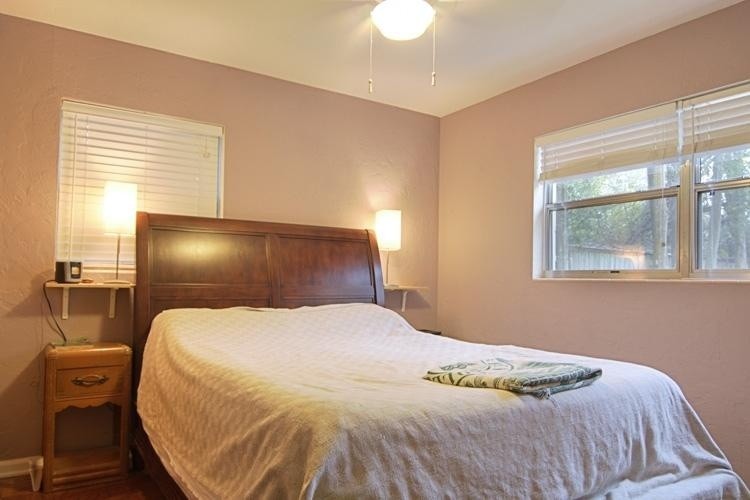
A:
[41, 340, 133, 495]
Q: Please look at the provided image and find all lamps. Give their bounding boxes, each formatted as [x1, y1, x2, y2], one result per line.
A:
[97, 179, 140, 286]
[372, 209, 404, 291]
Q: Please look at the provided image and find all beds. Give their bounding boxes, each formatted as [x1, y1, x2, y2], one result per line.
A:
[131, 210, 747, 499]
